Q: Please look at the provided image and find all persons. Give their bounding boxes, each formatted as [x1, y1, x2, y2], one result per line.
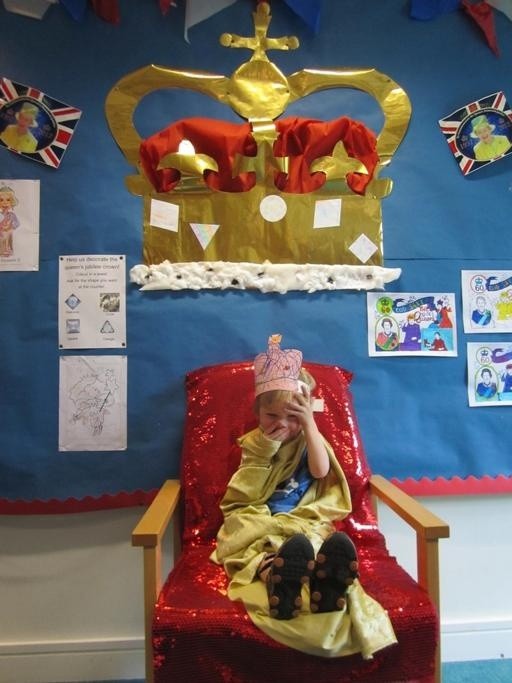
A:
[220, 386, 360, 621]
[0, 102, 39, 153]
[470, 115, 511, 161]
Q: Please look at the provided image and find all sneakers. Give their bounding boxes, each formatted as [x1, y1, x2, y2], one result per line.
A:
[311, 530, 359, 612]
[265, 534, 314, 619]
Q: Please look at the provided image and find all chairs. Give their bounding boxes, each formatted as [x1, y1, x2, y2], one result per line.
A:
[130, 361, 451, 683]
